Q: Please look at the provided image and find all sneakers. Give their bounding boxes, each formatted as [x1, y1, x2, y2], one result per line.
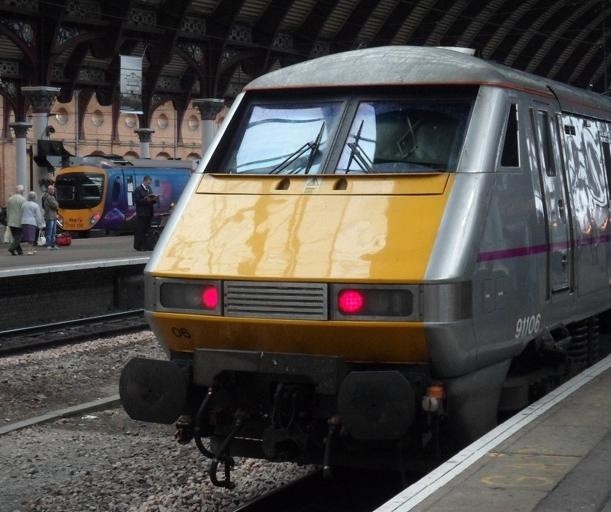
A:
[8, 246, 61, 255]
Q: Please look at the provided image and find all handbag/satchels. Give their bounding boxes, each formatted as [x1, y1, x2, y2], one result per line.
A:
[56, 234, 71, 245]
[37, 231, 46, 246]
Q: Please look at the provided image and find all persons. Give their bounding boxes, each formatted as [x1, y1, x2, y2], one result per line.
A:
[19, 190, 44, 256]
[132, 175, 153, 251]
[4, 184, 25, 256]
[42, 185, 59, 249]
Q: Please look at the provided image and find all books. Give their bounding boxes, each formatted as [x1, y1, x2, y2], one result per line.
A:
[147, 193, 160, 201]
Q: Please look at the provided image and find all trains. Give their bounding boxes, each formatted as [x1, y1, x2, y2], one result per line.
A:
[119, 45, 611, 490]
[45, 151, 203, 238]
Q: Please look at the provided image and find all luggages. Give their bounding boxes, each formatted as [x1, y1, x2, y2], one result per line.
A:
[135, 222, 163, 250]
